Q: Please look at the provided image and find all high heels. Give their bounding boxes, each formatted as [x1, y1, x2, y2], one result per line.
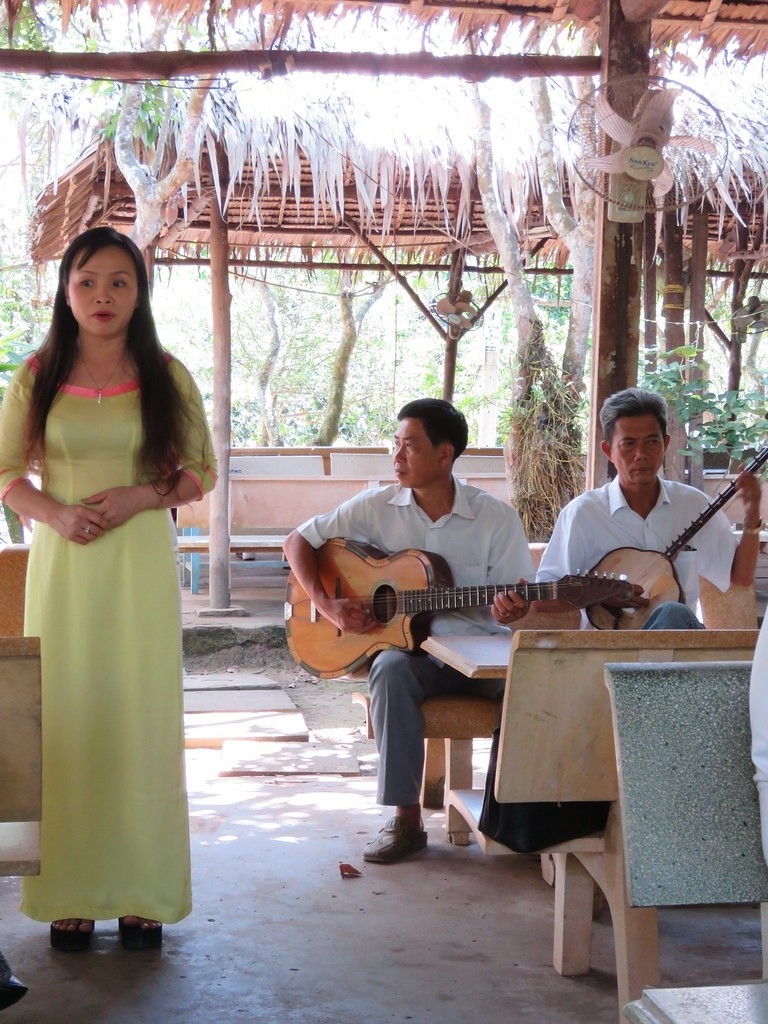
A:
[50, 918, 95, 949]
[119, 918, 162, 949]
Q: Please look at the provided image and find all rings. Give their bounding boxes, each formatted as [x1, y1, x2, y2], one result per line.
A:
[84, 524, 90, 533]
[500, 613, 510, 617]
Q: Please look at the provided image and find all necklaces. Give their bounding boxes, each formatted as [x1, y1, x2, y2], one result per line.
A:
[78, 349, 125, 404]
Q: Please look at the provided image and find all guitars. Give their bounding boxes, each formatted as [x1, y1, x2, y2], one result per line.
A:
[282, 535, 635, 682]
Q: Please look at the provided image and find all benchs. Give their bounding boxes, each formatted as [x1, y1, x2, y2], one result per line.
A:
[0, 544, 43, 876]
[343, 611, 768, 1024]
[177, 533, 292, 595]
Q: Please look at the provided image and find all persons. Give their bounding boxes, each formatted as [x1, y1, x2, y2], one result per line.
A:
[0, 226, 219, 949]
[748, 605, 767, 868]
[283, 399, 542, 862]
[533, 387, 764, 628]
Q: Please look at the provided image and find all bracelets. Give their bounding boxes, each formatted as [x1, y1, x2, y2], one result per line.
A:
[743, 516, 762, 534]
[153, 483, 164, 508]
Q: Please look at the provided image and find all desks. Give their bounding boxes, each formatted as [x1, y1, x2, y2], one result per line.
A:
[420, 633, 511, 680]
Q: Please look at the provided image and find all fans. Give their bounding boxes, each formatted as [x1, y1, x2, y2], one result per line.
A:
[432, 289, 485, 340]
[730, 296, 768, 344]
[566, 73, 730, 224]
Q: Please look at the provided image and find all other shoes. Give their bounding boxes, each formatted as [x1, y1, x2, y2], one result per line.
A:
[363, 817, 428, 862]
